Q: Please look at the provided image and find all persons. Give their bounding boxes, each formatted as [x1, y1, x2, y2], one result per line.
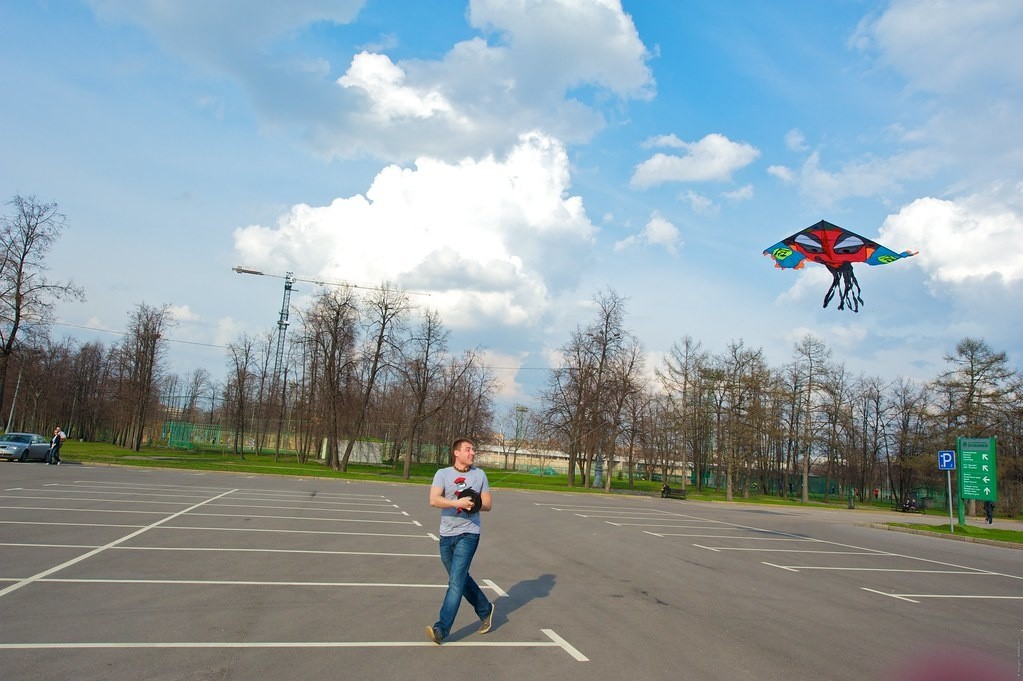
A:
[848, 486, 855, 509]
[902, 498, 919, 512]
[778, 481, 782, 496]
[45, 426, 67, 466]
[661, 483, 671, 498]
[874, 488, 879, 499]
[856, 488, 859, 495]
[984, 501, 995, 524]
[788, 481, 793, 494]
[425, 439, 495, 643]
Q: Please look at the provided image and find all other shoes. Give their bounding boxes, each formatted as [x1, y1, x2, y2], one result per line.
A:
[45, 463, 50, 466]
[57, 461, 61, 466]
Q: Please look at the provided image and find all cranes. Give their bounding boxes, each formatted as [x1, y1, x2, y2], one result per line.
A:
[231, 263, 432, 404]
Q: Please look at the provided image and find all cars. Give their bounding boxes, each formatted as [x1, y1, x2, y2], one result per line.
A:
[0, 433, 51, 462]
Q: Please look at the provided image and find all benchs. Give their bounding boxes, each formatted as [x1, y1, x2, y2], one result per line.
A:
[891, 504, 926, 515]
[668, 489, 686, 500]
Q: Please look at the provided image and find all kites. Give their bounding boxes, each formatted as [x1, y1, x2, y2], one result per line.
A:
[762, 219, 920, 313]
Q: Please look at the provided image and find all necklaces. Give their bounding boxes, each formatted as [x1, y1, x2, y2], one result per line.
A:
[454, 466, 470, 472]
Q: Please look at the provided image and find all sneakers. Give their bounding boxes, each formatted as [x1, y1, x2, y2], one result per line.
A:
[477, 604, 495, 633]
[425, 625, 443, 646]
